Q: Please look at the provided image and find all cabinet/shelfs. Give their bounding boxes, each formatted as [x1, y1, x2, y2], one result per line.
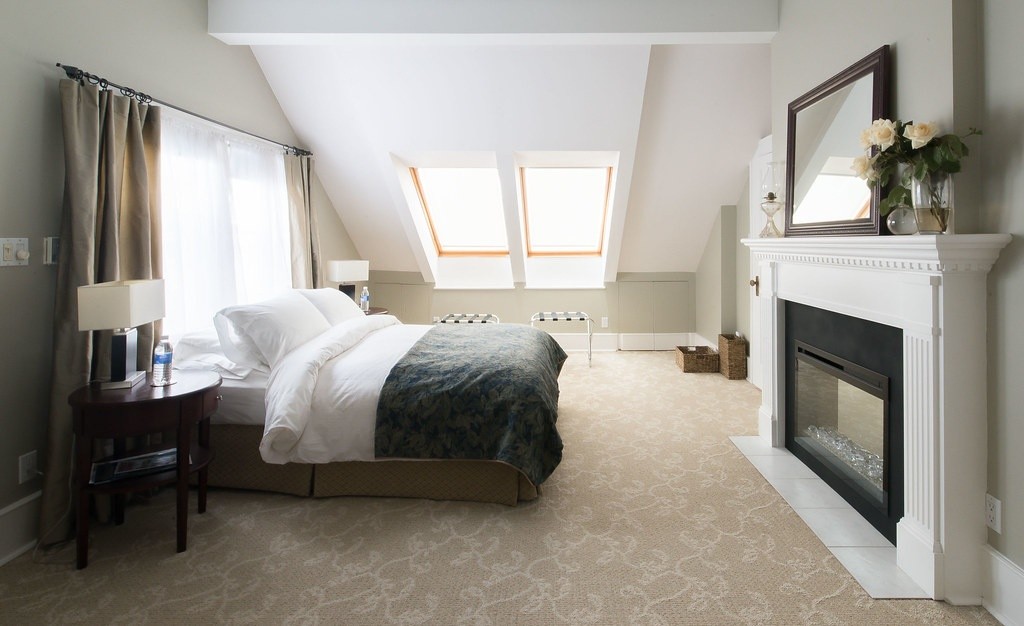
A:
[604, 271, 696, 336]
[370, 284, 431, 325]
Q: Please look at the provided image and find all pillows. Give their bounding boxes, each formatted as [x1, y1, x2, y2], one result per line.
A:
[297, 287, 366, 326]
[212, 291, 332, 374]
[172, 327, 257, 379]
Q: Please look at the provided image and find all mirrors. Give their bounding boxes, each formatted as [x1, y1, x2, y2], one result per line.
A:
[783, 44, 890, 237]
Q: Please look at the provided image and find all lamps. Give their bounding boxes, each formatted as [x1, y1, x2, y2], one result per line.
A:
[77, 280, 165, 390]
[327, 259, 370, 302]
[759, 163, 787, 238]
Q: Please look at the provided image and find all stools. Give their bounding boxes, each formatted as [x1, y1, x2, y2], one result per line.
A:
[438, 314, 499, 324]
[530, 310, 595, 363]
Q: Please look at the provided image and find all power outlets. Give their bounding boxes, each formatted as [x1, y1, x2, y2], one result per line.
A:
[18, 450, 40, 485]
[983, 493, 1001, 534]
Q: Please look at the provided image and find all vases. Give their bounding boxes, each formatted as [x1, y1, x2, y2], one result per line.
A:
[887, 202, 919, 234]
[910, 163, 953, 234]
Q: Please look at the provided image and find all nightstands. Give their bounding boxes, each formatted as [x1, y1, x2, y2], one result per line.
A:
[68, 371, 223, 571]
[364, 307, 389, 315]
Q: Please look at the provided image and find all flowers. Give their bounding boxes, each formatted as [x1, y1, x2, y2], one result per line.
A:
[850, 119, 983, 216]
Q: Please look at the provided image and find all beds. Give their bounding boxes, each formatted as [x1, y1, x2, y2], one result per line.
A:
[168, 325, 569, 506]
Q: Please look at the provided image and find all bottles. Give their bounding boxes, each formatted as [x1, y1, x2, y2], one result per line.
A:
[152, 335, 173, 386]
[360, 286, 370, 311]
